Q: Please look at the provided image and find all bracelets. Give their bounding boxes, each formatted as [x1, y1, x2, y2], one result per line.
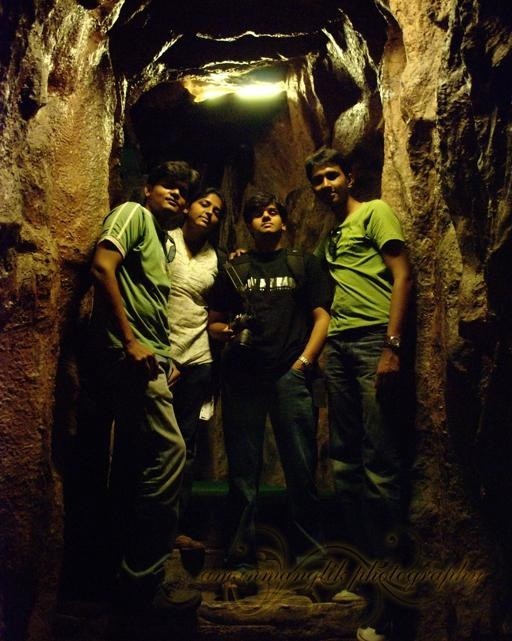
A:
[299, 353, 311, 368]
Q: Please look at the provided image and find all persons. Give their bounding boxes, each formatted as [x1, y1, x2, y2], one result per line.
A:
[164, 189, 225, 478]
[229, 146, 414, 523]
[90, 160, 202, 617]
[204, 189, 335, 594]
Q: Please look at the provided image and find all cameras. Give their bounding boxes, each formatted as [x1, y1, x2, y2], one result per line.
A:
[229, 312, 257, 351]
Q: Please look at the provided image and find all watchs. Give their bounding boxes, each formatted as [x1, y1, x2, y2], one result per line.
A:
[384, 333, 404, 346]
